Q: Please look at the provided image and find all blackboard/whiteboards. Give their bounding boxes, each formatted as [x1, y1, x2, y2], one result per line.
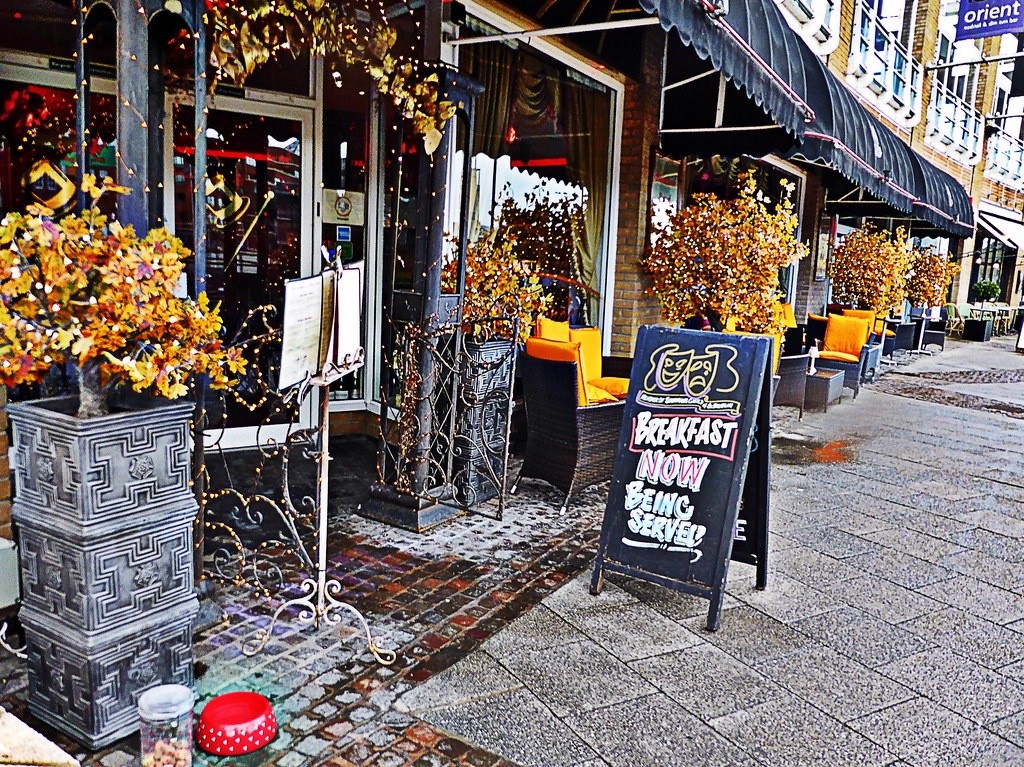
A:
[598, 323, 773, 600]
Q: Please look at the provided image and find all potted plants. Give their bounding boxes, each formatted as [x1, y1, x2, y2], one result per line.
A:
[962, 278, 1001, 342]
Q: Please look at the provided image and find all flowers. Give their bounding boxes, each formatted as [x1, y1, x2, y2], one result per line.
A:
[443, 233, 555, 347]
[0, 173, 253, 415]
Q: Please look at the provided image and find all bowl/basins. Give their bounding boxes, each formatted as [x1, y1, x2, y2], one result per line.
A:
[195, 692, 277, 755]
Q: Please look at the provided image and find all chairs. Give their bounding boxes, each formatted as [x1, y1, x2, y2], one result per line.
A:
[774, 354, 811, 421]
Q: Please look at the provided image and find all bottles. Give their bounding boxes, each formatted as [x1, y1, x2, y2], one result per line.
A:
[808, 339, 819, 375]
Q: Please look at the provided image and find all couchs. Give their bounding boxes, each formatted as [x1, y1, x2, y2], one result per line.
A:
[805, 302, 963, 401]
[535, 315, 634, 401]
[512, 338, 628, 516]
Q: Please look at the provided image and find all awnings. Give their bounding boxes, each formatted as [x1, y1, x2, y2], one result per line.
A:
[630, 0, 975, 240]
[981, 212, 1024, 255]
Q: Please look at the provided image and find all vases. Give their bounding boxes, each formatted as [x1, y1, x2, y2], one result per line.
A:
[7, 395, 201, 754]
[441, 341, 515, 506]
